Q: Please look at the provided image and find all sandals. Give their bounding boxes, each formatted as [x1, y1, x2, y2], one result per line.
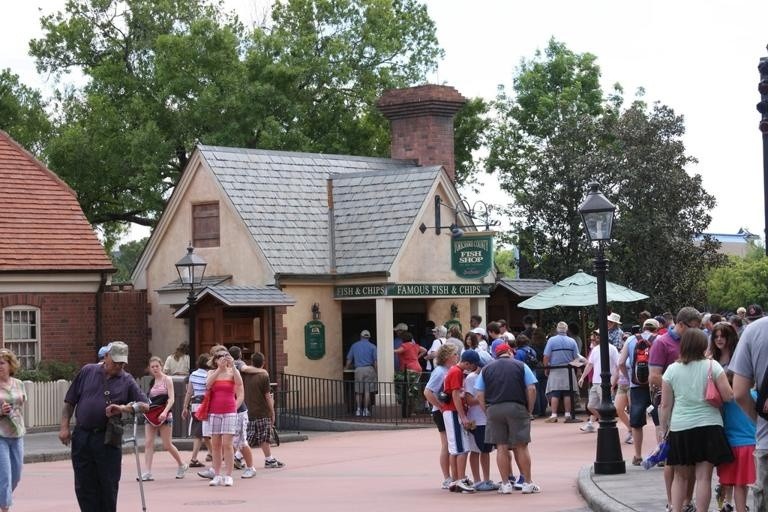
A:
[187, 458, 205, 469]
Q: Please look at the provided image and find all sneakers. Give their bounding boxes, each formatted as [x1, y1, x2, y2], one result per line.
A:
[135, 470, 157, 482]
[623, 431, 634, 444]
[233, 455, 245, 470]
[355, 407, 372, 417]
[542, 417, 558, 423]
[174, 462, 189, 479]
[196, 466, 236, 489]
[263, 456, 288, 470]
[239, 464, 259, 479]
[563, 416, 574, 423]
[579, 421, 599, 432]
[205, 452, 213, 463]
[633, 455, 666, 467]
[441, 474, 542, 495]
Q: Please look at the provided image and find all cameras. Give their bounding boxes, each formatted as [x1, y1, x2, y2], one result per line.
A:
[8, 410, 16, 420]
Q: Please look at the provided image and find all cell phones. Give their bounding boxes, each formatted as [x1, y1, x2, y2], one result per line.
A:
[224, 352, 229, 360]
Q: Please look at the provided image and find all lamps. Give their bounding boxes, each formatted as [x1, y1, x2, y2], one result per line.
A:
[451, 304, 461, 319]
[311, 301, 323, 320]
[420, 221, 466, 238]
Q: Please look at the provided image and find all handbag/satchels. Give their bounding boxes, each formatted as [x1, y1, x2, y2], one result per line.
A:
[438, 390, 453, 404]
[102, 414, 125, 449]
[705, 377, 725, 408]
[194, 387, 214, 422]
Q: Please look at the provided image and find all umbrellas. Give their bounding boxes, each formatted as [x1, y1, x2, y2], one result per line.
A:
[515, 266, 650, 359]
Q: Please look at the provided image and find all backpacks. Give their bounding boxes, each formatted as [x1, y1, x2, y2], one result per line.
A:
[632, 337, 652, 386]
[525, 351, 538, 371]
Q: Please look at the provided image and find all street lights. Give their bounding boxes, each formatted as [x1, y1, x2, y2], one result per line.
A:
[578, 181, 629, 473]
[176, 240, 208, 380]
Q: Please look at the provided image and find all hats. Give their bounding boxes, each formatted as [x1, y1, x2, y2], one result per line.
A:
[393, 323, 410, 330]
[96, 342, 112, 360]
[642, 318, 661, 329]
[106, 340, 129, 363]
[468, 327, 485, 336]
[497, 319, 507, 328]
[495, 342, 518, 356]
[460, 348, 486, 368]
[607, 312, 623, 326]
[360, 329, 372, 338]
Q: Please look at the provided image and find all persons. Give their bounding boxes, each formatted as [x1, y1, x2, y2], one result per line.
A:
[59, 341, 150, 512]
[0, 345, 29, 512]
[137, 357, 189, 480]
[516, 315, 586, 423]
[578, 313, 675, 465]
[182, 344, 284, 485]
[650, 306, 767, 511]
[394, 315, 515, 413]
[344, 330, 376, 417]
[424, 344, 541, 494]
[164, 343, 190, 376]
